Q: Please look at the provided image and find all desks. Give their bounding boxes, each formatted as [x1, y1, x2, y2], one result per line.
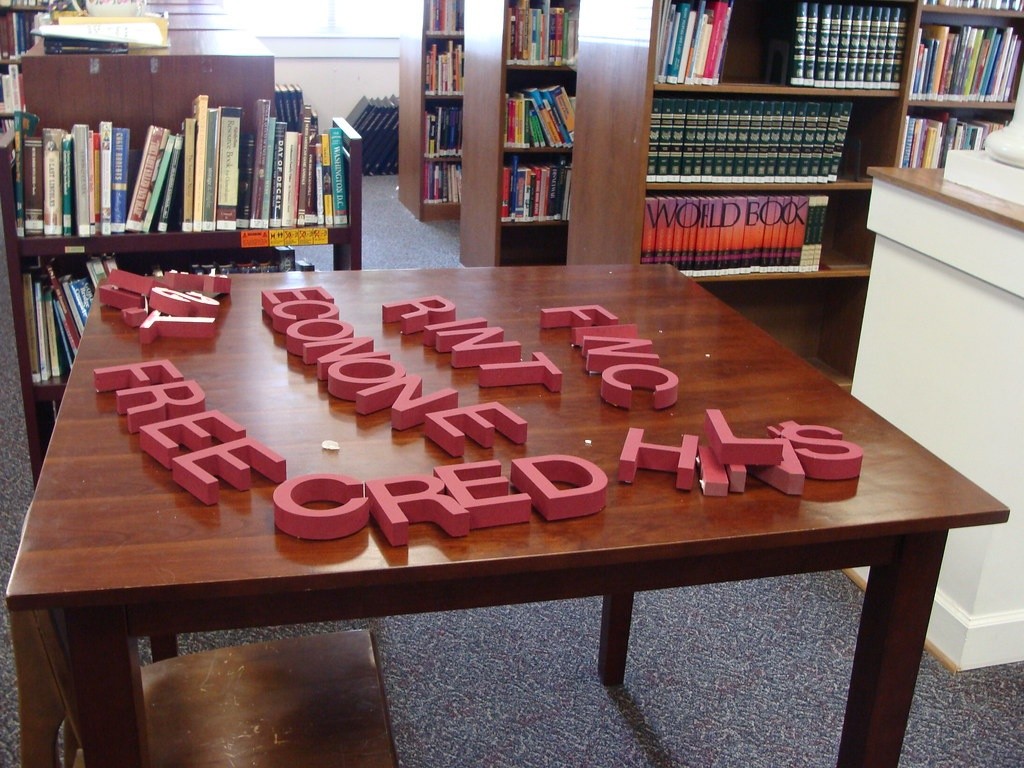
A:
[3, 263, 1007, 768]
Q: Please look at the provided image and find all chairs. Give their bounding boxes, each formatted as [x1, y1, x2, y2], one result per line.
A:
[7, 597, 397, 768]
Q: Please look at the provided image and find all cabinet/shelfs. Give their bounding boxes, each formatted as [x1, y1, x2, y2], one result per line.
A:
[398, 0, 463, 223]
[0, 0, 363, 485]
[567, 0, 1024, 392]
[459, 0, 580, 267]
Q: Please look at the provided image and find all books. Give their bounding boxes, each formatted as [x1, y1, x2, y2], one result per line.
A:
[898, 111, 1009, 168]
[275, 84, 399, 177]
[22, 253, 118, 385]
[145, 246, 316, 276]
[424, 0, 464, 204]
[0, 0, 128, 132]
[501, 1, 577, 223]
[13, 95, 349, 237]
[654, 0, 733, 85]
[759, 1, 909, 90]
[641, 194, 829, 278]
[909, 0, 1024, 102]
[646, 97, 854, 184]
